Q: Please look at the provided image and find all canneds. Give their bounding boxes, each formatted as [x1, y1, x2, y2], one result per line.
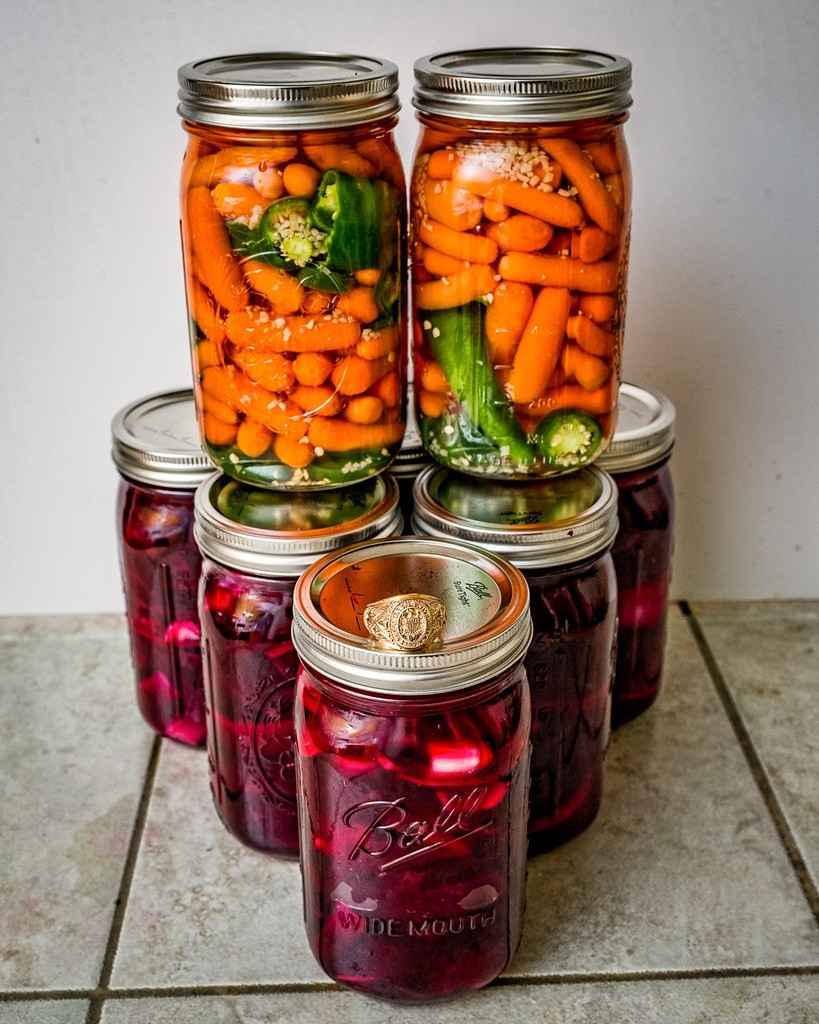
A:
[108, 45, 681, 1006]
